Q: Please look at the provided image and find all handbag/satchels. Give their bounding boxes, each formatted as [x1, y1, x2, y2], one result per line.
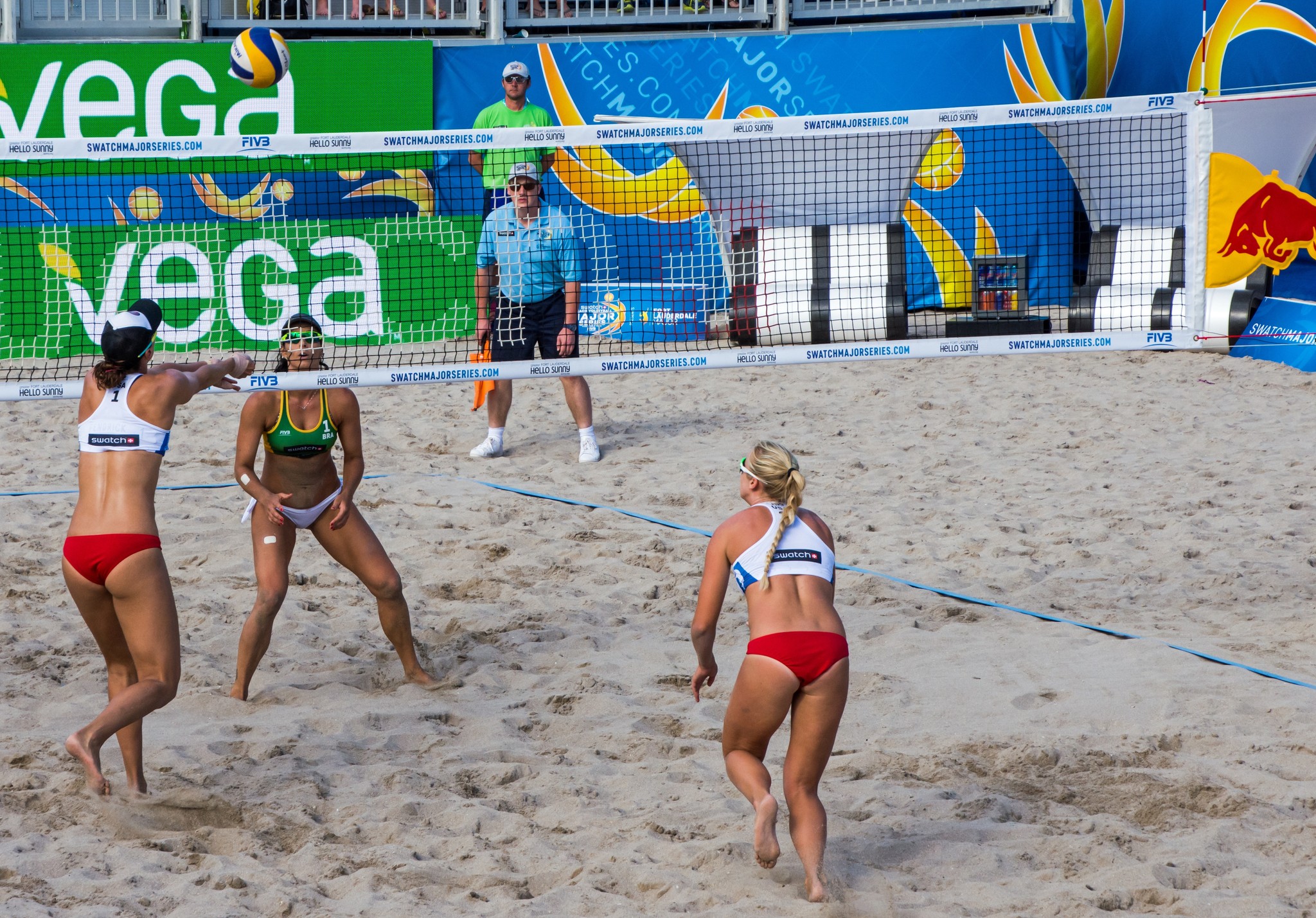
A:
[246, 0, 260, 16]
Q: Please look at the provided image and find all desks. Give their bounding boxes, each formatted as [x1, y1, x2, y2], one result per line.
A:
[572, 277, 712, 341]
[941, 312, 1060, 343]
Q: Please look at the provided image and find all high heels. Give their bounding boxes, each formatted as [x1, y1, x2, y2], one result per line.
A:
[721, 0, 740, 8]
[703, 0, 713, 9]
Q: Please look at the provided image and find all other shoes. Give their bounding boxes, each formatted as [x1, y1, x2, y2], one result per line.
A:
[481, 7, 488, 14]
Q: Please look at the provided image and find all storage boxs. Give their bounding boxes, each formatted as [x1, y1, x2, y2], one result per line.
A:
[970, 254, 1030, 320]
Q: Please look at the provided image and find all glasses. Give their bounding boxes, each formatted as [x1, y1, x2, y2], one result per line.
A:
[503, 76, 529, 83]
[739, 457, 768, 485]
[510, 183, 535, 191]
[280, 331, 323, 344]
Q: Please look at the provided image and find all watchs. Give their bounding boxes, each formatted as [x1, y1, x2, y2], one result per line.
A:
[563, 323, 577, 333]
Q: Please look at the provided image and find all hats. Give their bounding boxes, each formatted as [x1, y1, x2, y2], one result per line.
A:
[281, 313, 322, 336]
[509, 162, 540, 183]
[502, 60, 530, 78]
[101, 298, 163, 363]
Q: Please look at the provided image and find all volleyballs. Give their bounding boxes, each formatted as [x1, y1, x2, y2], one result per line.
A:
[229, 26, 290, 89]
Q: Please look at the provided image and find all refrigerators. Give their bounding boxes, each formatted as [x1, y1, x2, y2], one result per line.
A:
[971, 254, 1029, 321]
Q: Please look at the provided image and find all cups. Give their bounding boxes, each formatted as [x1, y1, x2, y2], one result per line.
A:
[480, 31, 486, 37]
[512, 29, 528, 38]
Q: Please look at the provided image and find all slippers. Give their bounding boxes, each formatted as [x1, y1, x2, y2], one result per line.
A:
[426, 4, 447, 20]
[387, 5, 405, 16]
[525, 7, 546, 18]
[362, 4, 374, 15]
[558, 7, 573, 17]
[377, 6, 388, 15]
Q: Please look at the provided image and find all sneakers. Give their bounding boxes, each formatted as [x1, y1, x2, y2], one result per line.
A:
[578, 437, 600, 463]
[683, 0, 708, 12]
[617, 0, 634, 12]
[469, 437, 504, 458]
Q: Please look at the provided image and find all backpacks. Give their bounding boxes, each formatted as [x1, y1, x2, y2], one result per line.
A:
[256, 0, 311, 39]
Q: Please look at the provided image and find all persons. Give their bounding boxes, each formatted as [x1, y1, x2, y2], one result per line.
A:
[469, 162, 601, 463]
[689, 441, 852, 901]
[617, 0, 739, 11]
[228, 313, 439, 703]
[62, 296, 255, 801]
[315, 0, 574, 20]
[465, 60, 557, 226]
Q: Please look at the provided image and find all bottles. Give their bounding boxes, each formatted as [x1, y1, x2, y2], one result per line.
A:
[179, 4, 188, 39]
